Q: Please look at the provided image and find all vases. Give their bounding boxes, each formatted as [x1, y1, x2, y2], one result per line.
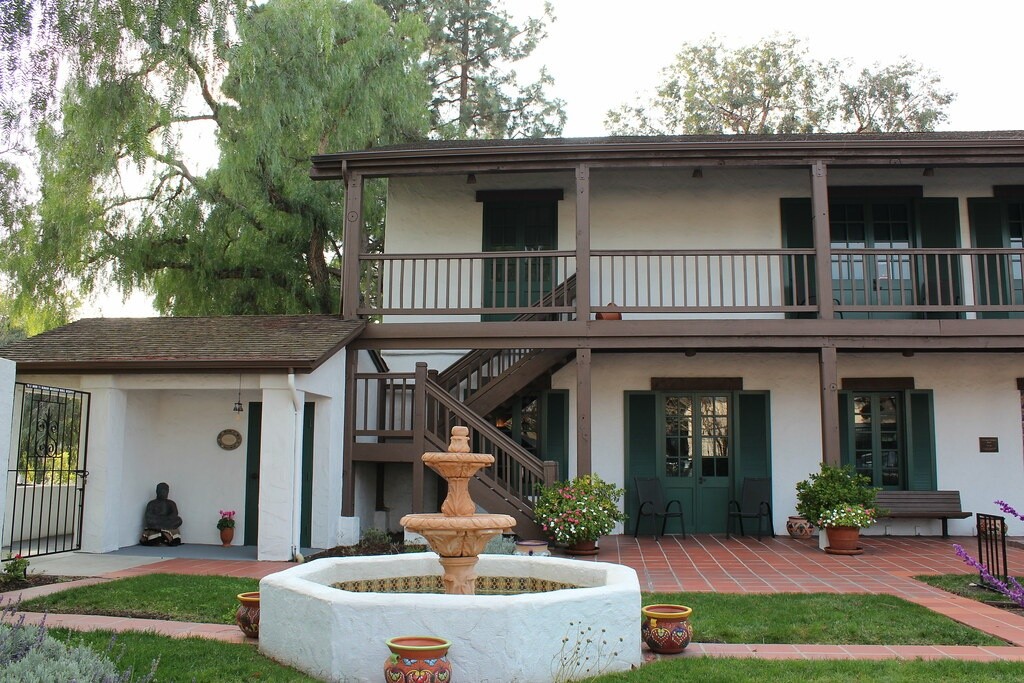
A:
[385, 638, 452, 683]
[565, 538, 599, 555]
[640, 605, 692, 654]
[819, 528, 830, 550]
[234, 591, 260, 637]
[823, 525, 864, 555]
[787, 516, 814, 540]
[220, 526, 233, 548]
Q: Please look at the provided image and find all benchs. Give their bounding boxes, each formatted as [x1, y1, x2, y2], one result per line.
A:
[874, 491, 971, 539]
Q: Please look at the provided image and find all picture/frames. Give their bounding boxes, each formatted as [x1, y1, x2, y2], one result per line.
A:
[986, 534, 1003, 540]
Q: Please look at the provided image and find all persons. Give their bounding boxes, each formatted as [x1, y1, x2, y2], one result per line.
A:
[140, 483, 182, 546]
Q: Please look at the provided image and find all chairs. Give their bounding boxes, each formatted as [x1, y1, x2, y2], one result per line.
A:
[635, 474, 686, 541]
[726, 477, 775, 540]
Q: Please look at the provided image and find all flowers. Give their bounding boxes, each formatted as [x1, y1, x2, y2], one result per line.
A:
[980, 519, 1009, 537]
[532, 473, 630, 546]
[818, 501, 878, 527]
[217, 510, 237, 530]
[796, 461, 891, 530]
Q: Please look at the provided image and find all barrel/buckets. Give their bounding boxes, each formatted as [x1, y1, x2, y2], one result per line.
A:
[596, 303, 622, 320]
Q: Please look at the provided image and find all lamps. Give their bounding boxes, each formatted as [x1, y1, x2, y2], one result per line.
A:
[233, 373, 243, 412]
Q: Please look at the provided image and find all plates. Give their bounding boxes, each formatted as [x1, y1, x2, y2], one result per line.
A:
[217, 429, 242, 450]
